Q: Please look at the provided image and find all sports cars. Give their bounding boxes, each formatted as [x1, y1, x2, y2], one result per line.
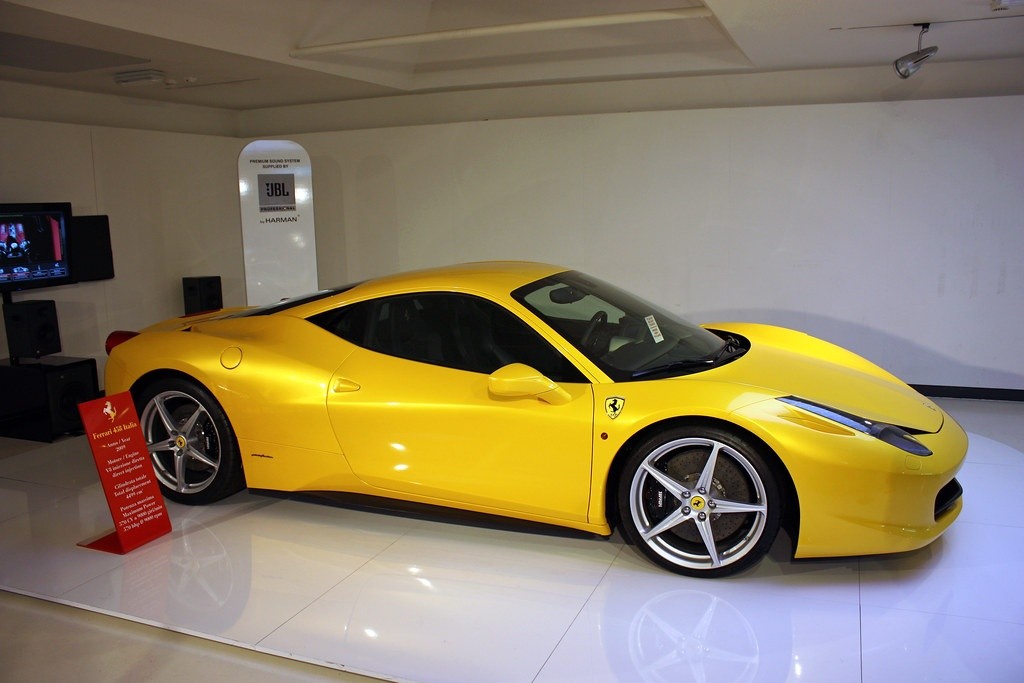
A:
[103, 260, 969, 579]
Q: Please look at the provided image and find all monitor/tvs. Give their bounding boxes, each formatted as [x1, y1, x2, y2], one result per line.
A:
[1, 202, 77, 292]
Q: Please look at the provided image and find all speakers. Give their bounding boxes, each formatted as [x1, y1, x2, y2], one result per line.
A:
[11, 299, 61, 360]
[182, 277, 224, 315]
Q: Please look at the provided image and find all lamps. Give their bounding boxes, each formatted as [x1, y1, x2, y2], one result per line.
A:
[892, 23, 939, 80]
